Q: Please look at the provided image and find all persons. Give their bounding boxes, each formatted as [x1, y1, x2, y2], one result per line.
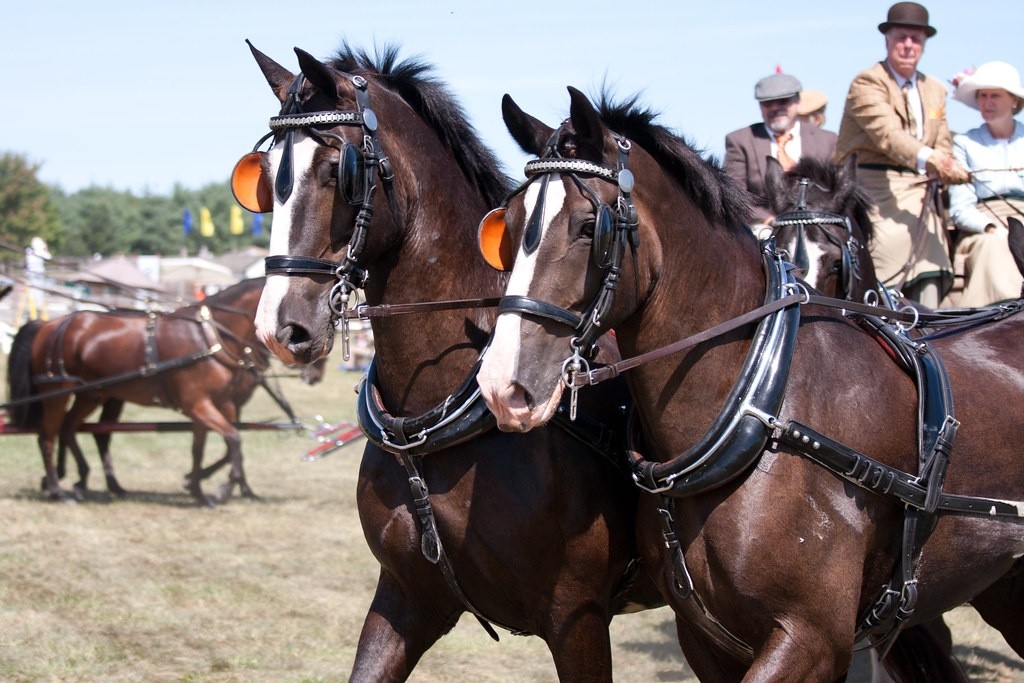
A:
[830, 2, 970, 310]
[723, 73, 839, 240]
[24, 236, 51, 324]
[939, 62, 1024, 311]
[796, 87, 828, 129]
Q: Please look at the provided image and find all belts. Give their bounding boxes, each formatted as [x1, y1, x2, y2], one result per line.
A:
[858, 163, 913, 171]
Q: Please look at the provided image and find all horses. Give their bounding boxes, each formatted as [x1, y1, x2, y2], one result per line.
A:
[472, 86, 1024, 683]
[243, 36, 957, 683]
[6, 276, 328, 505]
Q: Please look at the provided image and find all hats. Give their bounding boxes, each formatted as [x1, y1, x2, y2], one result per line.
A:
[878, 2, 937, 37]
[947, 61, 1024, 110]
[755, 74, 802, 101]
[798, 90, 827, 115]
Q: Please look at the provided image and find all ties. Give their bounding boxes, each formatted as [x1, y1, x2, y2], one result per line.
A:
[774, 134, 797, 172]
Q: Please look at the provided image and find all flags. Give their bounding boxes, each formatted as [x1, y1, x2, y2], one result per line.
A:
[252, 212, 263, 236]
[201, 205, 214, 238]
[183, 207, 192, 237]
[230, 204, 244, 235]
